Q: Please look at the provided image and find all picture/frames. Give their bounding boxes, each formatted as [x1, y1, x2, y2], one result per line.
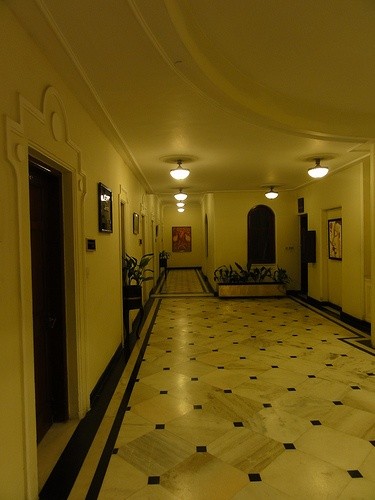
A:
[328, 218, 342, 261]
[98, 182, 113, 234]
[172, 226, 191, 253]
[133, 212, 139, 235]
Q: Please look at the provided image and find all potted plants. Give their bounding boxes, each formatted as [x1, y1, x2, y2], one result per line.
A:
[121, 252, 154, 310]
[159, 250, 171, 268]
[213, 261, 289, 300]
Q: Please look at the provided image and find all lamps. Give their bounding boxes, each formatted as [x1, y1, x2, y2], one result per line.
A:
[174, 189, 188, 212]
[265, 186, 279, 200]
[308, 159, 329, 179]
[169, 160, 190, 180]
